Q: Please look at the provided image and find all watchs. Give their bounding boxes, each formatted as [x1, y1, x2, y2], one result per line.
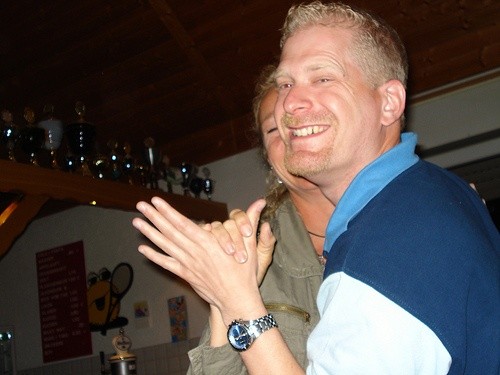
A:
[226, 314, 278, 351]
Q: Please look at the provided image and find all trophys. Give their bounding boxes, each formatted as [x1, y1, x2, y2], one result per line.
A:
[0, 109, 19, 163]
[39, 103, 65, 168]
[64, 100, 96, 177]
[18, 105, 46, 167]
[89, 129, 214, 200]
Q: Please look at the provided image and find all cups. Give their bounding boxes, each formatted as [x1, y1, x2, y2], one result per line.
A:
[0, 98, 216, 201]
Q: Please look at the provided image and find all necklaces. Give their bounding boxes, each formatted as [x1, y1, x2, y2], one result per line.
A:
[308, 230, 327, 238]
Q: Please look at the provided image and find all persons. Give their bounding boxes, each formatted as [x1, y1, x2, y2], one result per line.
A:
[184, 62, 337, 375]
[132, 0, 500, 375]
